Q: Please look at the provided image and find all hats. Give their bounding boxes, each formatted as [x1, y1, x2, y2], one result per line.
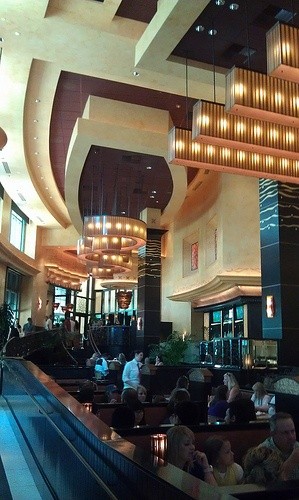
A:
[104, 384, 121, 397]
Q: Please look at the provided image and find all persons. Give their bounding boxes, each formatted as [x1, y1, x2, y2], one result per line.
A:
[80, 380, 146, 429]
[45, 314, 53, 331]
[23, 318, 33, 335]
[107, 320, 120, 326]
[242, 411, 299, 483]
[208, 372, 272, 424]
[90, 317, 106, 326]
[162, 426, 245, 486]
[86, 352, 126, 394]
[155, 355, 164, 366]
[140, 357, 149, 369]
[17, 323, 21, 333]
[169, 376, 190, 402]
[8, 325, 19, 339]
[122, 350, 144, 390]
[56, 323, 60, 327]
[64, 316, 81, 332]
[158, 389, 200, 432]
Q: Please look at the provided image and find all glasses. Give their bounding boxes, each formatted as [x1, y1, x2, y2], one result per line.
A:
[225, 373, 231, 378]
[109, 389, 120, 393]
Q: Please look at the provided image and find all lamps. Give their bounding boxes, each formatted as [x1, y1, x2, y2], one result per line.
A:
[46, 300, 50, 307]
[153, 455, 168, 467]
[166, 0, 299, 184]
[52, 302, 75, 313]
[76, 176, 148, 281]
[82, 402, 94, 414]
[150, 433, 168, 461]
[115, 289, 133, 309]
[36, 296, 43, 312]
[266, 295, 275, 318]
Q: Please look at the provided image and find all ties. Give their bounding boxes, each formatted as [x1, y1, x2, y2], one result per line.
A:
[137, 363, 141, 375]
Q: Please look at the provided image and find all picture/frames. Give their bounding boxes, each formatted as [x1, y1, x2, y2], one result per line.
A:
[191, 241, 199, 271]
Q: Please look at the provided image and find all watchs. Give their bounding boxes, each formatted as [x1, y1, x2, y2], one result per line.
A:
[204, 465, 213, 472]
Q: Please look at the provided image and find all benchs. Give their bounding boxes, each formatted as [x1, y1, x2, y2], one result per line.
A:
[52, 377, 299, 500]
